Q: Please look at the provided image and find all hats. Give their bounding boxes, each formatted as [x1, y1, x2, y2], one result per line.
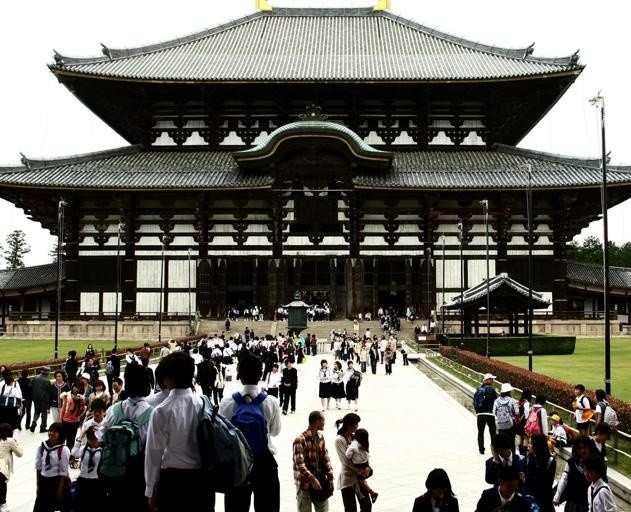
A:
[549, 414, 561, 423]
[483, 373, 497, 381]
[79, 372, 90, 382]
[500, 382, 516, 394]
[41, 366, 51, 374]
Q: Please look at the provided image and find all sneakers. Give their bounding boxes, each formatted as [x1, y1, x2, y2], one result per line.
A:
[40, 428, 49, 433]
[30, 423, 36, 432]
[371, 493, 378, 503]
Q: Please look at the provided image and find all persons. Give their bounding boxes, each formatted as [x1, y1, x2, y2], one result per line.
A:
[523, 394, 549, 452]
[292, 410, 334, 512]
[273, 301, 328, 322]
[345, 429, 378, 503]
[144, 365, 172, 405]
[579, 456, 618, 512]
[334, 413, 372, 512]
[515, 389, 532, 452]
[328, 302, 438, 375]
[330, 361, 346, 409]
[593, 389, 610, 425]
[472, 373, 498, 454]
[221, 304, 269, 331]
[550, 412, 573, 446]
[474, 470, 535, 512]
[217, 353, 283, 511]
[343, 361, 361, 412]
[572, 384, 592, 436]
[521, 433, 556, 512]
[549, 414, 568, 456]
[588, 422, 611, 474]
[144, 352, 217, 512]
[316, 360, 333, 412]
[551, 435, 597, 512]
[485, 434, 527, 487]
[159, 331, 318, 415]
[0, 343, 155, 511]
[492, 383, 520, 453]
[412, 468, 459, 511]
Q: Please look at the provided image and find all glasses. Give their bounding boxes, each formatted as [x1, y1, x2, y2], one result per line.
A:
[112, 384, 119, 387]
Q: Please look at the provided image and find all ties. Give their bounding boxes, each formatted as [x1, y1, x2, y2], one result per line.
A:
[504, 458, 509, 468]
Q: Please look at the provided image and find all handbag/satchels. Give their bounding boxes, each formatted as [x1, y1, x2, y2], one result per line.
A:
[515, 413, 521, 430]
[1, 395, 21, 409]
[308, 471, 333, 509]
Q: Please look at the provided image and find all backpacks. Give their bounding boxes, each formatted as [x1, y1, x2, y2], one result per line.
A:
[599, 399, 619, 432]
[230, 391, 267, 455]
[105, 360, 114, 377]
[524, 406, 543, 437]
[581, 396, 596, 418]
[472, 386, 492, 409]
[96, 400, 152, 480]
[195, 394, 254, 494]
[497, 397, 513, 429]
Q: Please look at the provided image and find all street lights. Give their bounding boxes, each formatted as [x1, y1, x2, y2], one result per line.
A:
[458, 222, 470, 345]
[589, 93, 612, 395]
[441, 232, 449, 341]
[185, 246, 193, 341]
[53, 197, 68, 363]
[477, 197, 495, 362]
[522, 162, 538, 374]
[156, 236, 169, 348]
[111, 220, 128, 355]
[424, 244, 434, 333]
